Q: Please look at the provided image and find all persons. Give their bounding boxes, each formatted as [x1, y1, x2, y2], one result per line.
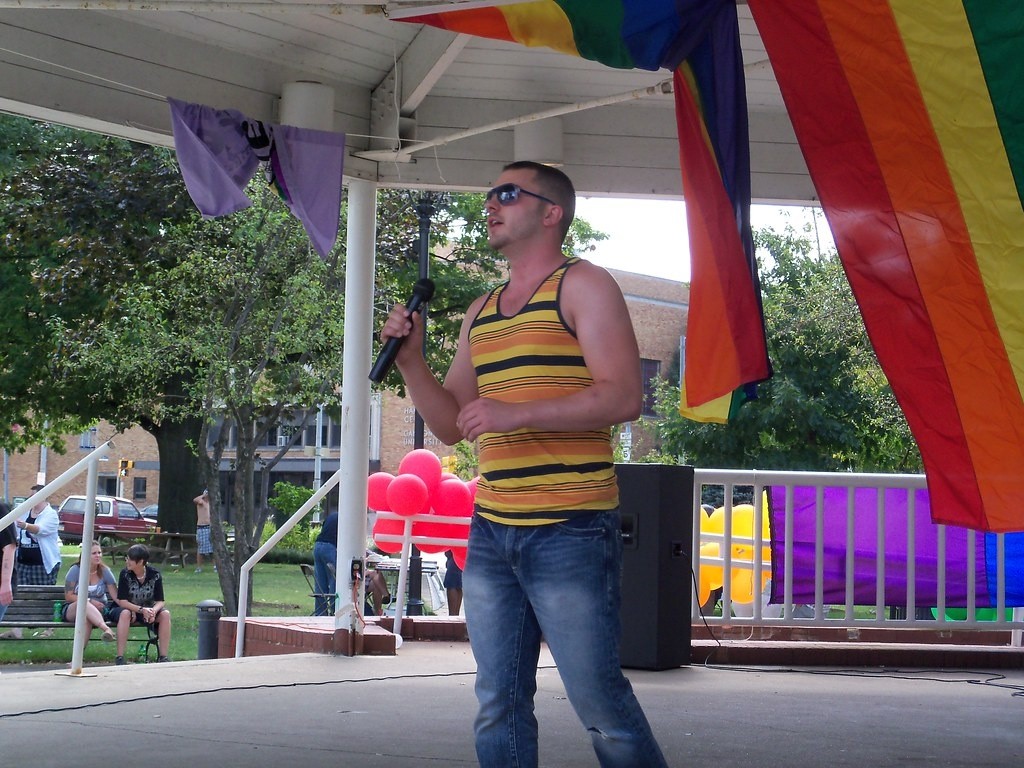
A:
[365, 548, 396, 616]
[381, 161, 669, 768]
[0, 484, 63, 640]
[443, 550, 463, 616]
[107, 543, 172, 665]
[0, 499, 17, 622]
[192, 487, 218, 572]
[60, 539, 136, 650]
[313, 511, 338, 616]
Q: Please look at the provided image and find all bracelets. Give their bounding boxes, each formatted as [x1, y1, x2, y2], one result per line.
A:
[139, 607, 143, 614]
[25, 523, 28, 529]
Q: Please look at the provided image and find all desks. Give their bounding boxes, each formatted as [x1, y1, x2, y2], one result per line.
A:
[94, 528, 198, 567]
[375, 566, 437, 610]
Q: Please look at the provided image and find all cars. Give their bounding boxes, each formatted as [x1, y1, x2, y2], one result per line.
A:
[57, 494, 158, 543]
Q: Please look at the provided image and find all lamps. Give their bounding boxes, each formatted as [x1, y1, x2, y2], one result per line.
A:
[278, 435, 285, 446]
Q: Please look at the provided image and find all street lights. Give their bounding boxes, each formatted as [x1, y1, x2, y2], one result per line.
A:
[404, 189, 443, 615]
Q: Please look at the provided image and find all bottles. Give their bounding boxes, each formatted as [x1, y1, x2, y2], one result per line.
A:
[53, 601, 62, 622]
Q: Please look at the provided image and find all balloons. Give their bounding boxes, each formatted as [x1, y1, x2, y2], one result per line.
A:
[367, 448, 480, 570]
[697, 489, 773, 606]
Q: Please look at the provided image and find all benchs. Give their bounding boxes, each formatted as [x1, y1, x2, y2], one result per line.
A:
[102, 546, 196, 567]
[0, 584, 161, 663]
[221, 529, 236, 562]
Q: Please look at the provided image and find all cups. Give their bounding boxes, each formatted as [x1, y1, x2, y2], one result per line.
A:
[156, 527, 161, 534]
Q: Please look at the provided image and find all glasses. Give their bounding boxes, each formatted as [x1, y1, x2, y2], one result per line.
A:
[485, 183, 556, 206]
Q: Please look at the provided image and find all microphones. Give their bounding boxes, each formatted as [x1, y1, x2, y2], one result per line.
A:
[368, 278, 435, 384]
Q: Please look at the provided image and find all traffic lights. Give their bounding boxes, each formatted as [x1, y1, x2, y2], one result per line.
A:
[118, 460, 135, 477]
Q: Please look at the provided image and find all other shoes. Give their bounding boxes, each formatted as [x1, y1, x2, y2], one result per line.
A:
[115, 656, 125, 665]
[194, 567, 203, 573]
[213, 566, 218, 573]
[158, 656, 170, 663]
[382, 594, 396, 604]
[101, 627, 115, 642]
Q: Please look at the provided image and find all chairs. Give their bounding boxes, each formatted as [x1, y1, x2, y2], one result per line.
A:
[300, 563, 336, 616]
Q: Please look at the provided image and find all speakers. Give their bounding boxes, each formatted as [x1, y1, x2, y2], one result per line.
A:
[611, 463, 694, 672]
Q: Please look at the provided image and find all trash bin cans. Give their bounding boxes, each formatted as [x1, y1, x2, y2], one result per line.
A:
[12, 497, 27, 509]
